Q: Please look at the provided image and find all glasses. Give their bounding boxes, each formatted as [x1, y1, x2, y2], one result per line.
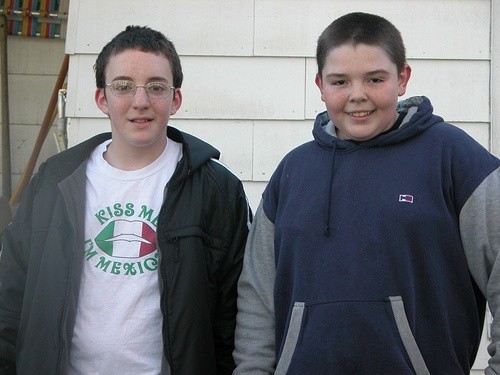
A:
[104, 80, 175, 100]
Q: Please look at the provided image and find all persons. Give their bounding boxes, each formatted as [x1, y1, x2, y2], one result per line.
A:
[0, 24, 254, 375]
[232, 12, 500, 375]
